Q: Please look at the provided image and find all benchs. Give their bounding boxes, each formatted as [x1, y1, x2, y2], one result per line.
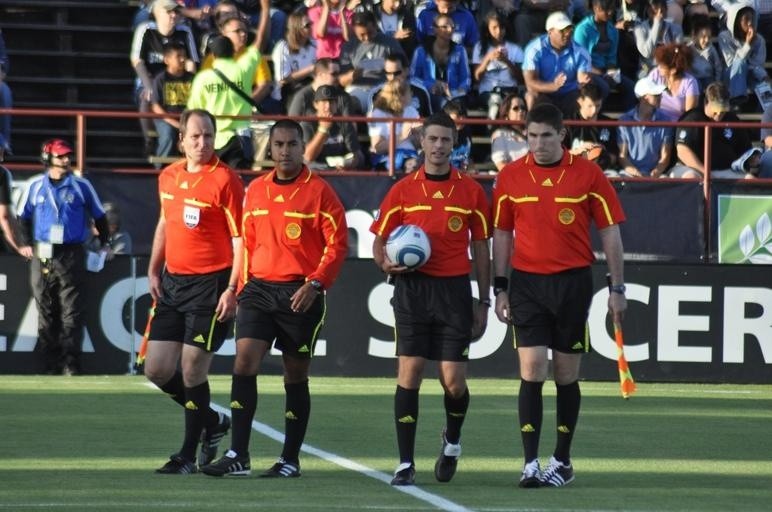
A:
[142, 0, 771, 183]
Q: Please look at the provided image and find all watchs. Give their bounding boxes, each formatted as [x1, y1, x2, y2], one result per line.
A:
[309, 279, 322, 291]
[479, 298, 492, 306]
[611, 284, 625, 294]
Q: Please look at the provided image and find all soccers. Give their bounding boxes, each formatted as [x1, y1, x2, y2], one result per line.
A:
[386, 224, 431, 269]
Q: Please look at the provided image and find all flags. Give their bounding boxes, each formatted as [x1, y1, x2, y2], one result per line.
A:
[612, 316, 636, 402]
[136, 299, 157, 365]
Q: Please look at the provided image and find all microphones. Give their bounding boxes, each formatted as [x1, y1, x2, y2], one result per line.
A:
[40, 160, 69, 170]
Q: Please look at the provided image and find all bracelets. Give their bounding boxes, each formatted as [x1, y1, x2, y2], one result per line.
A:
[377, 261, 385, 273]
[226, 284, 237, 293]
[493, 277, 510, 294]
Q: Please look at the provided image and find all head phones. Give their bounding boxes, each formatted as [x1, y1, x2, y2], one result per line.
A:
[39, 142, 51, 165]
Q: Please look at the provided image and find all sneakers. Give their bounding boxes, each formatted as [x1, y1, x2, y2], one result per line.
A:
[255, 453, 303, 479]
[433, 425, 464, 484]
[518, 454, 578, 491]
[388, 461, 417, 488]
[152, 453, 199, 477]
[198, 412, 252, 479]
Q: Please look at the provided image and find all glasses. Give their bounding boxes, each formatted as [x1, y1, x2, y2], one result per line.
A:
[513, 105, 526, 113]
[300, 20, 314, 29]
[383, 70, 404, 76]
[328, 72, 342, 77]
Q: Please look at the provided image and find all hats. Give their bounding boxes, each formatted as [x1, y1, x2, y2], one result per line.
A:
[544, 10, 575, 31]
[633, 76, 668, 99]
[42, 138, 74, 157]
[206, 34, 235, 58]
[314, 83, 339, 101]
[152, 0, 183, 13]
[705, 82, 733, 113]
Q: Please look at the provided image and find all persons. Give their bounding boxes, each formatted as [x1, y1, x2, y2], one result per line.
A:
[143, 109, 245, 475]
[0, 135, 34, 259]
[370, 112, 493, 484]
[88, 203, 132, 255]
[199, 120, 348, 477]
[1, 34, 13, 161]
[491, 103, 626, 488]
[15, 139, 107, 377]
